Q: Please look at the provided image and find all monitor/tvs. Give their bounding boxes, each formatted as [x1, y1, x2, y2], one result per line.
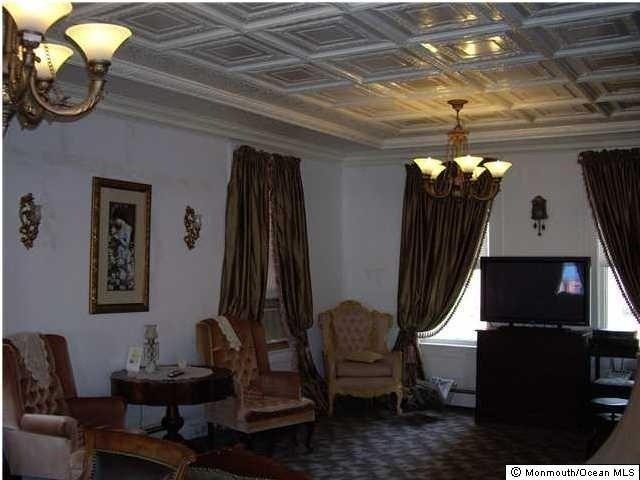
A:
[480, 255, 593, 327]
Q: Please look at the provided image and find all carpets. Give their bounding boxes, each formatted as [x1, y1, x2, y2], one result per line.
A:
[186, 443, 312, 480]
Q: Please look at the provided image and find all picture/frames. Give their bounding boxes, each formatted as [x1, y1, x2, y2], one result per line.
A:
[89, 176, 152, 314]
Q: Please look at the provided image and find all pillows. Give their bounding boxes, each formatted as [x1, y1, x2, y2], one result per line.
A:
[345, 351, 384, 363]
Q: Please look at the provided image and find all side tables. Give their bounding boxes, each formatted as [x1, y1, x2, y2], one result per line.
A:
[111, 364, 232, 443]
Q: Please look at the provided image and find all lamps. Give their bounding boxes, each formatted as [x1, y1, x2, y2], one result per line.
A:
[19, 193, 42, 250]
[531, 195, 548, 236]
[413, 99, 513, 202]
[2, 3, 134, 139]
[143, 323, 160, 373]
[183, 205, 204, 250]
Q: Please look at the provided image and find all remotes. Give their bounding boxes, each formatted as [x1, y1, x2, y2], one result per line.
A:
[168, 369, 184, 377]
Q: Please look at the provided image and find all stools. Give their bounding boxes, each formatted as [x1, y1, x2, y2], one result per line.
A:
[593, 397, 629, 439]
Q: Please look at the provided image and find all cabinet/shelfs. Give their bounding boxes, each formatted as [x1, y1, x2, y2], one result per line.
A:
[475, 330, 591, 429]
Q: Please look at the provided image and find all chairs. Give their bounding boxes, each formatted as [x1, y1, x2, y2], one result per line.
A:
[3, 332, 197, 480]
[318, 301, 403, 417]
[197, 316, 316, 450]
